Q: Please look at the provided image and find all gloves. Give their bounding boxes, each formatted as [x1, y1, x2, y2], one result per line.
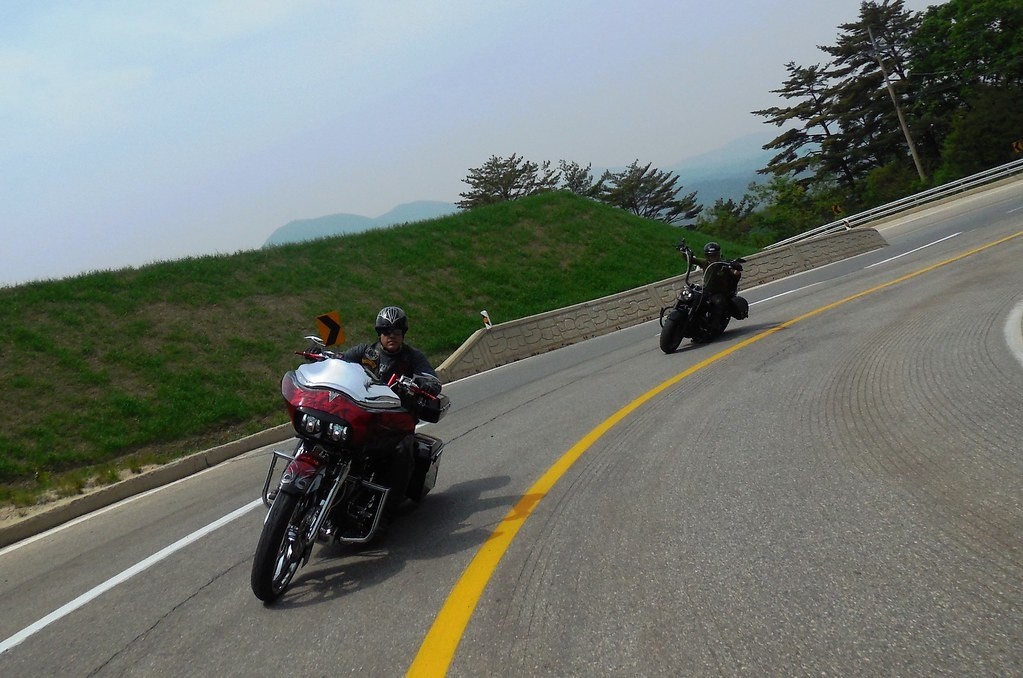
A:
[304, 346, 321, 361]
[420, 382, 441, 397]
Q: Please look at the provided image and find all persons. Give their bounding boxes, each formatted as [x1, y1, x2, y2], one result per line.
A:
[678, 240, 742, 332]
[303, 305, 440, 506]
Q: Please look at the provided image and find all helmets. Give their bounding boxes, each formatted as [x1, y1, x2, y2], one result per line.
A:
[375, 307, 408, 334]
[704, 243, 720, 254]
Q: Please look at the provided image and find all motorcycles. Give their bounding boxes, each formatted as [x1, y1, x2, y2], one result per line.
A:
[251, 336, 451, 605]
[658, 238, 750, 355]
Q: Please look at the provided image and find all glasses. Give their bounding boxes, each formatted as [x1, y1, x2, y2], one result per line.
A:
[379, 328, 402, 337]
